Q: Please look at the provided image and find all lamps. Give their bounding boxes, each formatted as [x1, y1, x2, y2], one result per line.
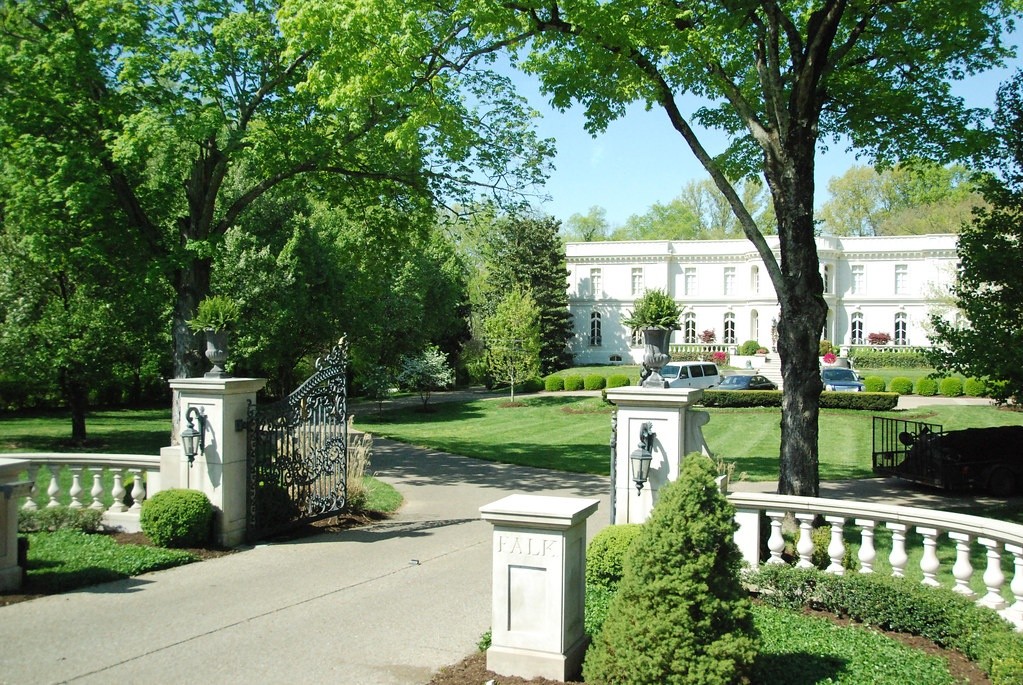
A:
[180, 406, 206, 468]
[630, 421, 657, 496]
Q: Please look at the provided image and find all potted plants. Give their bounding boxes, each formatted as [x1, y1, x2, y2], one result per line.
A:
[619, 287, 687, 389]
[184, 293, 244, 379]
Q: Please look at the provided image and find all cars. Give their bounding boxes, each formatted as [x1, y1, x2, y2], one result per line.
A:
[708, 375, 779, 391]
[820, 368, 867, 391]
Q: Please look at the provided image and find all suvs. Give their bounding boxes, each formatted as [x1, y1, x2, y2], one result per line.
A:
[658, 361, 720, 389]
